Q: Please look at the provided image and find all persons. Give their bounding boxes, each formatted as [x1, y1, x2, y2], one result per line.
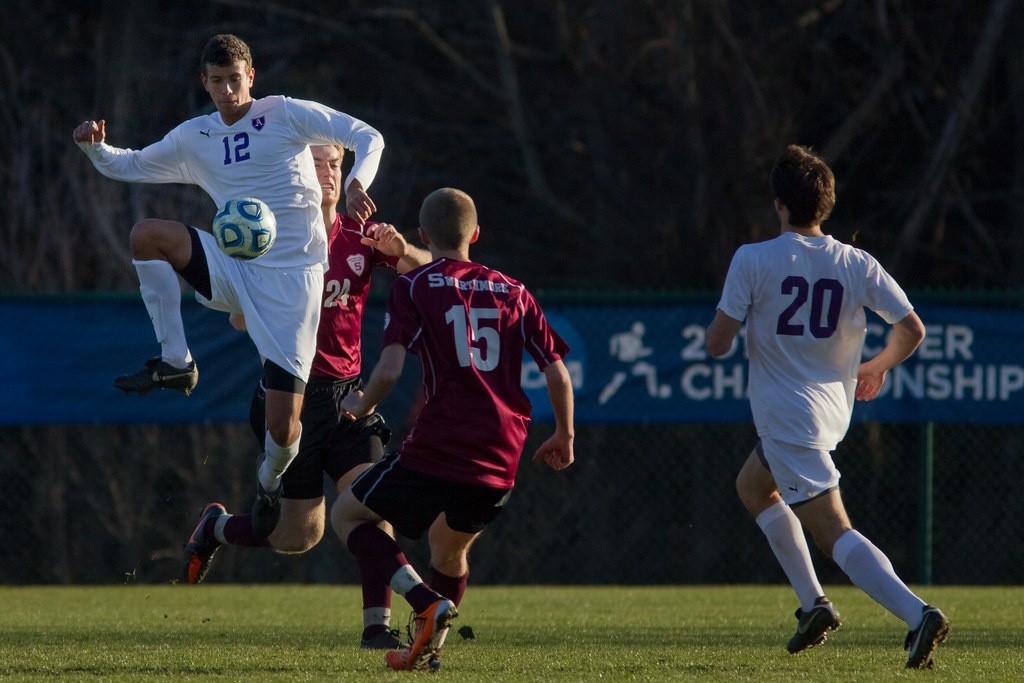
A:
[703, 144, 949, 669]
[330, 186, 575, 672]
[74, 33, 411, 648]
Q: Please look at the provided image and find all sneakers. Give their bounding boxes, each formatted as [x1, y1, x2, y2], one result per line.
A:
[113, 358, 198, 397]
[904, 604, 951, 669]
[254, 454, 284, 539]
[171, 502, 226, 583]
[386, 595, 459, 671]
[360, 629, 410, 651]
[427, 649, 442, 671]
[788, 596, 842, 654]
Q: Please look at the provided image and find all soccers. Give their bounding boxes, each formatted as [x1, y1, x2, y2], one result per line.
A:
[212, 196, 278, 261]
